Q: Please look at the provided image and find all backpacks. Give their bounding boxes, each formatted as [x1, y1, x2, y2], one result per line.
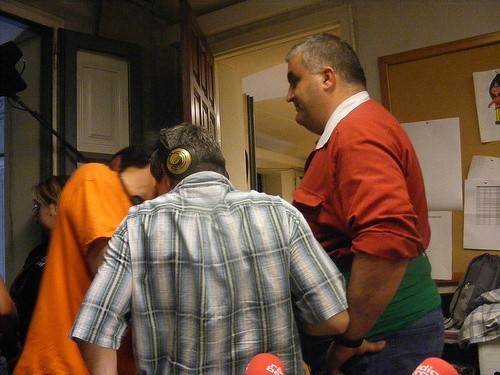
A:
[448, 251, 500, 331]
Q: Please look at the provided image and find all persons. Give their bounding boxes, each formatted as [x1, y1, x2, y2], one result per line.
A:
[12, 143, 156, 375]
[0, 174, 69, 375]
[67, 123, 349, 375]
[286, 32, 445, 375]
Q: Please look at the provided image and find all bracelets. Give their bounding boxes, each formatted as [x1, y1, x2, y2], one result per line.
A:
[336, 334, 365, 349]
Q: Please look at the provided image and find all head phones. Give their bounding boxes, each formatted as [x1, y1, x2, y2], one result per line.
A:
[155, 134, 199, 181]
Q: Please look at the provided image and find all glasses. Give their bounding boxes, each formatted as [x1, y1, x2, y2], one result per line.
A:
[32, 203, 53, 212]
